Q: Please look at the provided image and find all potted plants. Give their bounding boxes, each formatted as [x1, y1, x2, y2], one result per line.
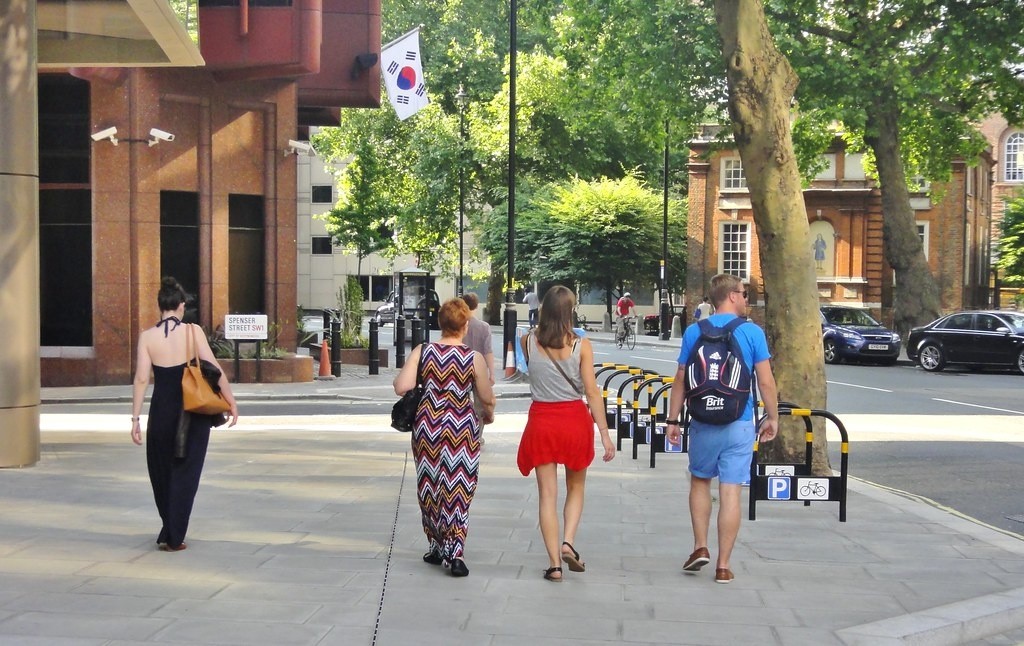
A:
[297, 304, 318, 348]
[309, 270, 388, 367]
[207, 318, 314, 382]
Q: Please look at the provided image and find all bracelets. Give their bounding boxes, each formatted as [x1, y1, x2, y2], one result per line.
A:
[666, 419, 678, 425]
[132, 417, 139, 421]
[600, 426, 608, 431]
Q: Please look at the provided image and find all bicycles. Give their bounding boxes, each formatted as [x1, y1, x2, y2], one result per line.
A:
[614, 316, 638, 350]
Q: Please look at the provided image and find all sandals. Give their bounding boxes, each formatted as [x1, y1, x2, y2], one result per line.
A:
[543, 567, 562, 582]
[561, 542, 586, 572]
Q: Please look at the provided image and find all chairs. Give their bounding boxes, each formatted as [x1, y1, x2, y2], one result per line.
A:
[843, 314, 852, 323]
[992, 316, 1005, 331]
[979, 318, 988, 329]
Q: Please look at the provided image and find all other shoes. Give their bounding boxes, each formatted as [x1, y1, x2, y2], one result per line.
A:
[451, 559, 469, 576]
[683, 547, 710, 570]
[423, 552, 444, 564]
[158, 542, 186, 551]
[529, 327, 533, 330]
[716, 568, 735, 584]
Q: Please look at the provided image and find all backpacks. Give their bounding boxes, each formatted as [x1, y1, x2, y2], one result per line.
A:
[684, 317, 751, 424]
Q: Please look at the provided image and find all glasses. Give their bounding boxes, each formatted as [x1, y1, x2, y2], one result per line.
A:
[625, 296, 630, 298]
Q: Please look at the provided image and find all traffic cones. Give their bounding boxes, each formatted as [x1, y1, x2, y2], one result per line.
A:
[314, 339, 338, 381]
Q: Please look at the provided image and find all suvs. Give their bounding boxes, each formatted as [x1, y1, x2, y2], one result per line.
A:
[643, 304, 686, 335]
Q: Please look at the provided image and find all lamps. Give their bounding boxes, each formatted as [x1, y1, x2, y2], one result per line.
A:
[350, 53, 378, 82]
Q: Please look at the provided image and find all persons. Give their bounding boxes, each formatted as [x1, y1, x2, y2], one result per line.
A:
[393, 298, 497, 576]
[666, 273, 779, 583]
[462, 292, 495, 446]
[130, 277, 238, 553]
[517, 286, 615, 581]
[523, 288, 539, 330]
[698, 296, 712, 321]
[615, 292, 637, 346]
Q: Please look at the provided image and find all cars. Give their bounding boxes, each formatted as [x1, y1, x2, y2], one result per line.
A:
[374, 288, 441, 331]
[820, 304, 902, 366]
[906, 309, 1024, 375]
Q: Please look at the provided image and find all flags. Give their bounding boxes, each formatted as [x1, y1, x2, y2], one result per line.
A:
[381, 30, 428, 121]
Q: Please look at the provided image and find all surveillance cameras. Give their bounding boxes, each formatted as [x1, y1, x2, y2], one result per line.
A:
[150, 128, 175, 141]
[303, 141, 316, 157]
[289, 139, 310, 151]
[91, 127, 117, 142]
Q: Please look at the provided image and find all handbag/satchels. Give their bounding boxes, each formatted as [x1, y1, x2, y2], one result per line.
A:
[391, 341, 427, 432]
[181, 323, 232, 415]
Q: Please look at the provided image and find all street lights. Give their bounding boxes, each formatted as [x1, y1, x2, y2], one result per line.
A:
[455, 79, 471, 298]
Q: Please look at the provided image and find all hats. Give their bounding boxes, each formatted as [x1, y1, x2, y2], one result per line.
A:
[624, 292, 630, 297]
